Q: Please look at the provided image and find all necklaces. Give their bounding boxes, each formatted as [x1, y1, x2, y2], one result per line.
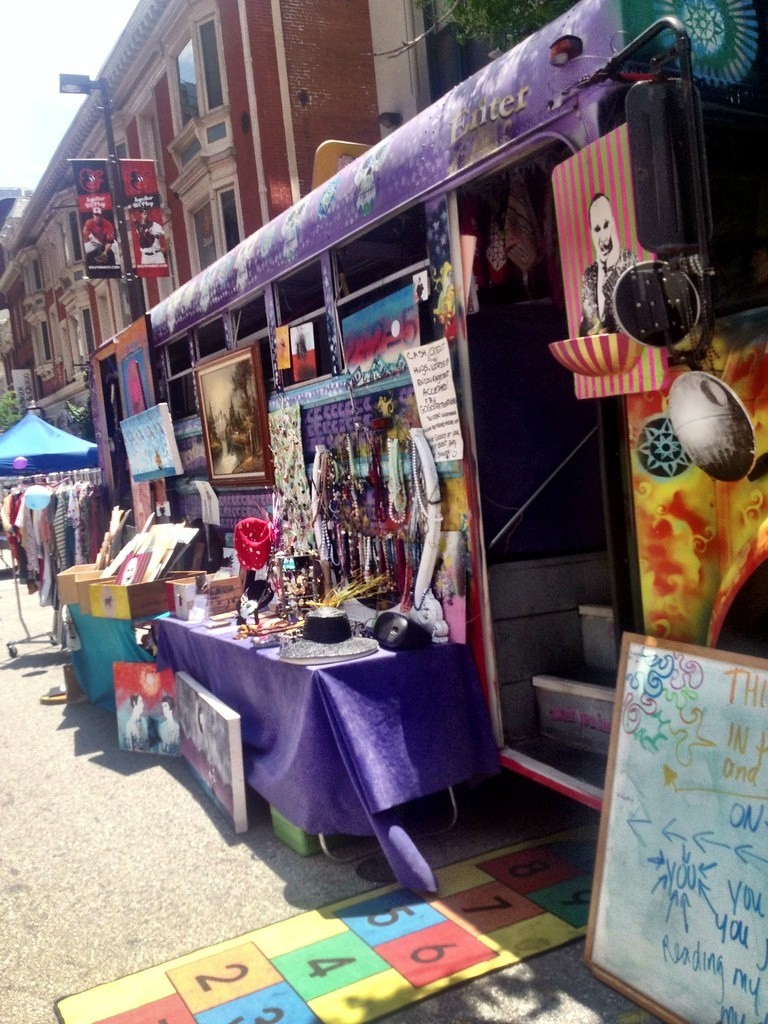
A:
[233, 418, 462, 653]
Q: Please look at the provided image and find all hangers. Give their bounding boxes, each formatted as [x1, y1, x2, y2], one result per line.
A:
[11, 466, 104, 491]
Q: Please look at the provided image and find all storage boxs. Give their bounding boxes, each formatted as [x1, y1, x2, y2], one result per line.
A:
[55, 561, 247, 621]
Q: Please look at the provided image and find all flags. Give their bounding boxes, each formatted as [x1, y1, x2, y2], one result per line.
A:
[66, 157, 125, 281]
[116, 157, 173, 278]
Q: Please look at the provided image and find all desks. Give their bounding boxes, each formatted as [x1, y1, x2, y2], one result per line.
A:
[63, 588, 506, 864]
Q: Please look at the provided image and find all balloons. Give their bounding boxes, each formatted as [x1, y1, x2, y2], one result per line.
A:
[24, 484, 52, 511]
[12, 455, 28, 470]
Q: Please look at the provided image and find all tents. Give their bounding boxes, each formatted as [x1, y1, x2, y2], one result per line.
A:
[0, 407, 105, 479]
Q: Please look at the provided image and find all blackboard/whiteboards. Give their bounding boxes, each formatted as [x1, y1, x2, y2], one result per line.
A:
[580, 631, 768, 1024]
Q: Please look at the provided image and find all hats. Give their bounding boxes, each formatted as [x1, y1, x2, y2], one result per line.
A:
[276, 605, 378, 658]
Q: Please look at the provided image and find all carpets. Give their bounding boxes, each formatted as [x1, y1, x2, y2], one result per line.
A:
[53, 827, 598, 1022]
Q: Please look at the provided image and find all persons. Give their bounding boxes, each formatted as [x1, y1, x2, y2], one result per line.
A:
[82, 205, 118, 266]
[579, 190, 643, 336]
[128, 202, 167, 267]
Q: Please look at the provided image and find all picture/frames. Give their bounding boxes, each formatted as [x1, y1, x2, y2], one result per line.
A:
[192, 338, 277, 487]
